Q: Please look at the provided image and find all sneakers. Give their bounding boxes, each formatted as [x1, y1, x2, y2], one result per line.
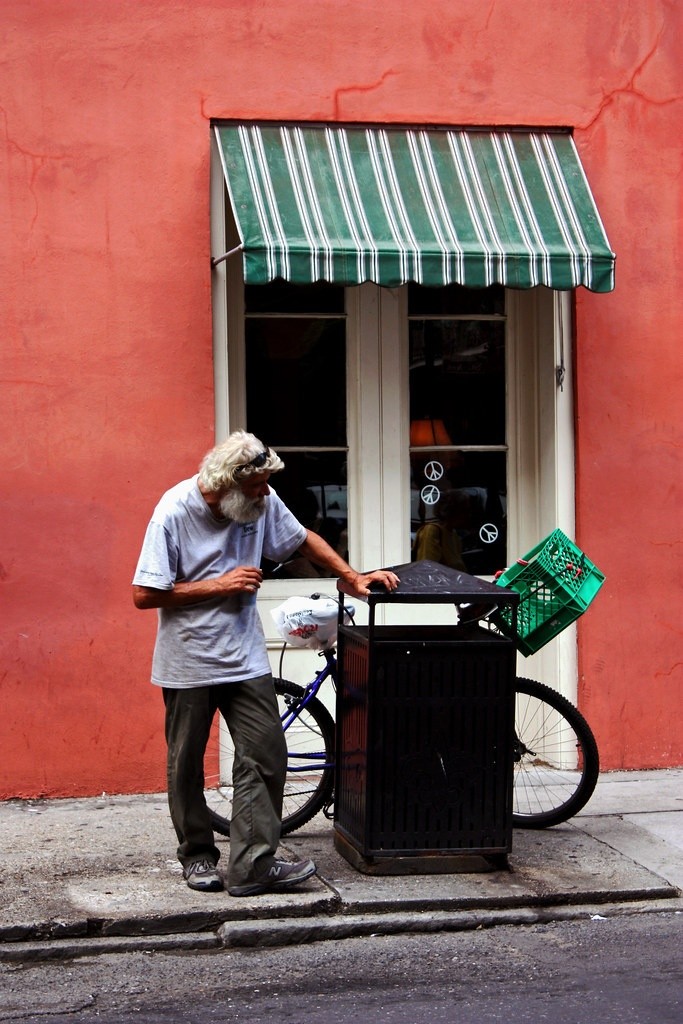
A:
[227, 859, 317, 897]
[182, 861, 224, 889]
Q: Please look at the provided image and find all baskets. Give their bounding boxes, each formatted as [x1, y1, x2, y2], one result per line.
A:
[489, 529, 605, 659]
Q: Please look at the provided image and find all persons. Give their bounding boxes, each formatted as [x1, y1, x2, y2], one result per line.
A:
[130, 428, 400, 897]
[276, 482, 347, 579]
[413, 492, 475, 574]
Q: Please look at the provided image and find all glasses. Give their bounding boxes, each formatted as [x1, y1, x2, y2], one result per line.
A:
[235, 444, 271, 471]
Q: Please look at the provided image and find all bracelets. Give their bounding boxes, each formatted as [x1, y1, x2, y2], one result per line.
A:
[352, 572, 358, 585]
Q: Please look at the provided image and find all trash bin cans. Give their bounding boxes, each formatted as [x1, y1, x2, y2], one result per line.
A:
[330, 560, 517, 877]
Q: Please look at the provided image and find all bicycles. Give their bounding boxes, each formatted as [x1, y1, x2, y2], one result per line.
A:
[202, 526, 607, 841]
